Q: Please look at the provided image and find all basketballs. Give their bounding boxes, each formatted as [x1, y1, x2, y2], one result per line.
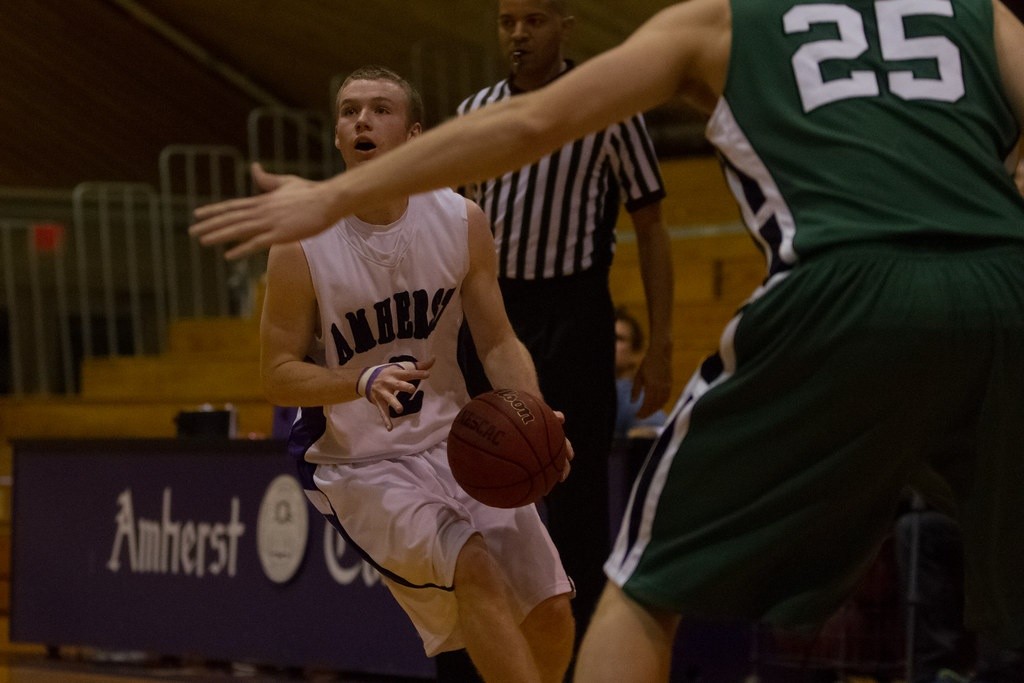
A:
[445, 389, 564, 511]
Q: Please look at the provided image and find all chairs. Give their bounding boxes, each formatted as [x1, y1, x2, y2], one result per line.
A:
[173, 405, 239, 436]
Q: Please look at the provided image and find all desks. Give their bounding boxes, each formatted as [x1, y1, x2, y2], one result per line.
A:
[8, 434, 441, 681]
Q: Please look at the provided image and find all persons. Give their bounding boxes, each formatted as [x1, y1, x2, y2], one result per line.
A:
[188, 0, 1023, 681]
[258, 69, 576, 682]
